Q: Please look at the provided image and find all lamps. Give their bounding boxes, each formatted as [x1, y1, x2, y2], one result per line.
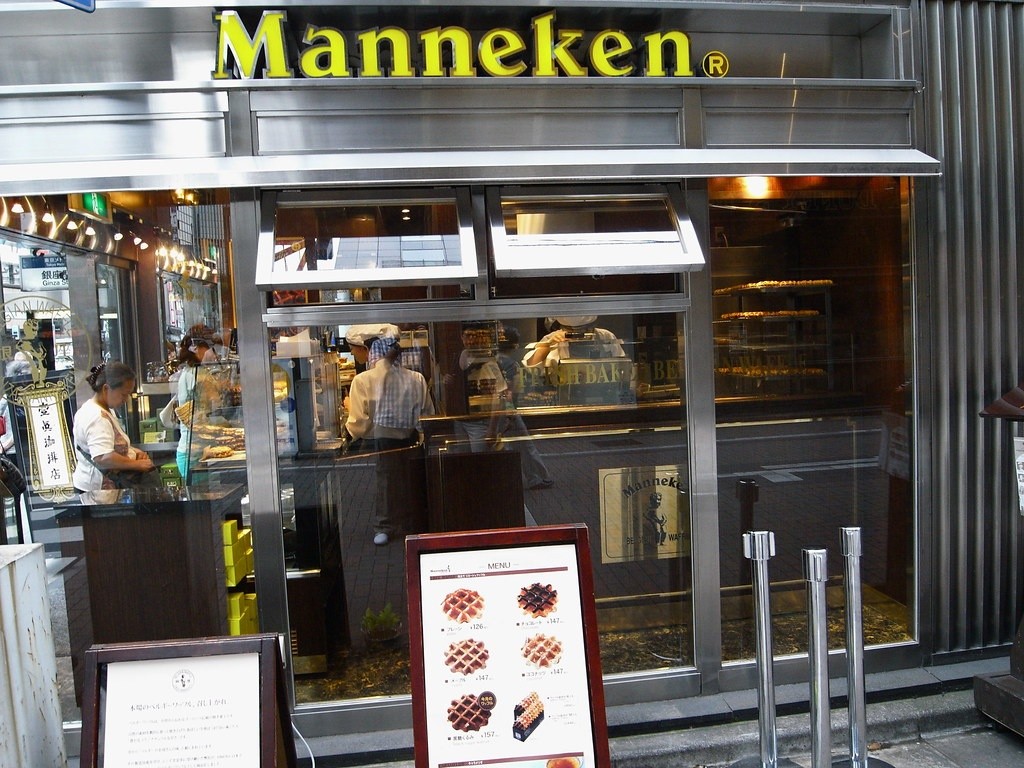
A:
[10, 197, 216, 277]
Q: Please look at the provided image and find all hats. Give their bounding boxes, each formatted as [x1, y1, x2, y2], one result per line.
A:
[346, 324, 402, 345]
[556, 315, 598, 327]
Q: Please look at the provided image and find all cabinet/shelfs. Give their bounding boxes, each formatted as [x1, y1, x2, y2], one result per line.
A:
[423, 433, 524, 535]
[52, 468, 251, 707]
[713, 284, 833, 404]
[184, 351, 346, 486]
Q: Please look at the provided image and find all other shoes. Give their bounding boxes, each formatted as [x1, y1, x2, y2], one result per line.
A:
[529, 479, 555, 491]
[374, 531, 389, 545]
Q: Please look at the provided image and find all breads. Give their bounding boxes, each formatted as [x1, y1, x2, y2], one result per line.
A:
[715, 280, 833, 377]
[204, 434, 245, 456]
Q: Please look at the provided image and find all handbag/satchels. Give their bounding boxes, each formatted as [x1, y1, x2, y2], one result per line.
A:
[159, 395, 181, 430]
[0, 441, 26, 497]
[115, 465, 173, 515]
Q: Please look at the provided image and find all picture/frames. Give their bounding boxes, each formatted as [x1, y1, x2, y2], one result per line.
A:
[4, 367, 77, 558]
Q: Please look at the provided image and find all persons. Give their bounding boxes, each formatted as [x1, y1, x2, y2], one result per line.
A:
[71, 361, 155, 497]
[199, 334, 234, 380]
[175, 324, 223, 482]
[344, 337, 437, 454]
[345, 322, 387, 372]
[439, 318, 556, 491]
[387, 323, 402, 344]
[0, 350, 37, 467]
[372, 323, 437, 547]
[521, 315, 637, 400]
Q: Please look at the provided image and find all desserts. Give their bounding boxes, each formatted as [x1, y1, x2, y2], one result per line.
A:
[440, 589, 484, 624]
[444, 639, 489, 675]
[518, 582, 558, 616]
[521, 634, 562, 668]
[514, 691, 544, 742]
[447, 694, 491, 731]
